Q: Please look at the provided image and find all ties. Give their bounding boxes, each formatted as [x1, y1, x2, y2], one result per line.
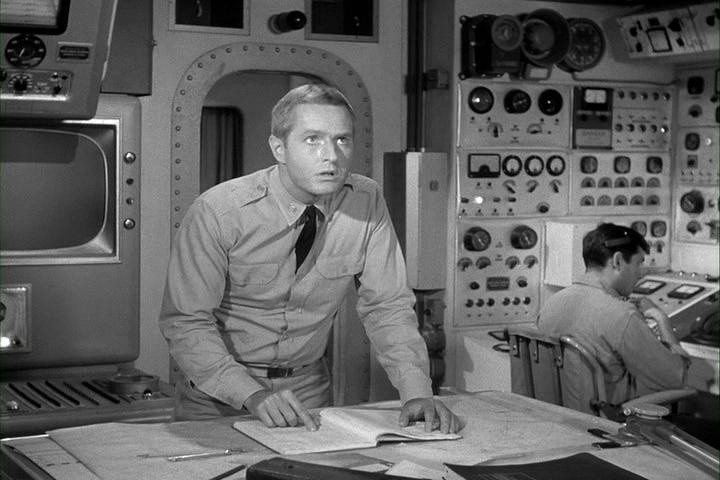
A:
[294, 206, 316, 272]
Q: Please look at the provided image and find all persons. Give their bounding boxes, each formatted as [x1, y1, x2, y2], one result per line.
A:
[535, 222, 691, 418]
[157, 85, 463, 436]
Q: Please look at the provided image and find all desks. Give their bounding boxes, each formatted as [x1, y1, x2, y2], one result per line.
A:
[0, 390, 720, 480]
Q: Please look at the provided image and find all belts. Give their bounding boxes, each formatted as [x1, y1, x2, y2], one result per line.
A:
[238, 359, 327, 379]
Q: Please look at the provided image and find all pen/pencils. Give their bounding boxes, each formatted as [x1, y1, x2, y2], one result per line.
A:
[136, 449, 243, 461]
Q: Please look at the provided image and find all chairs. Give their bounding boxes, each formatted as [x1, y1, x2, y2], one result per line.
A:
[503, 326, 699, 435]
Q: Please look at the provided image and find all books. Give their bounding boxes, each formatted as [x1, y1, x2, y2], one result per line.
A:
[231, 404, 461, 455]
